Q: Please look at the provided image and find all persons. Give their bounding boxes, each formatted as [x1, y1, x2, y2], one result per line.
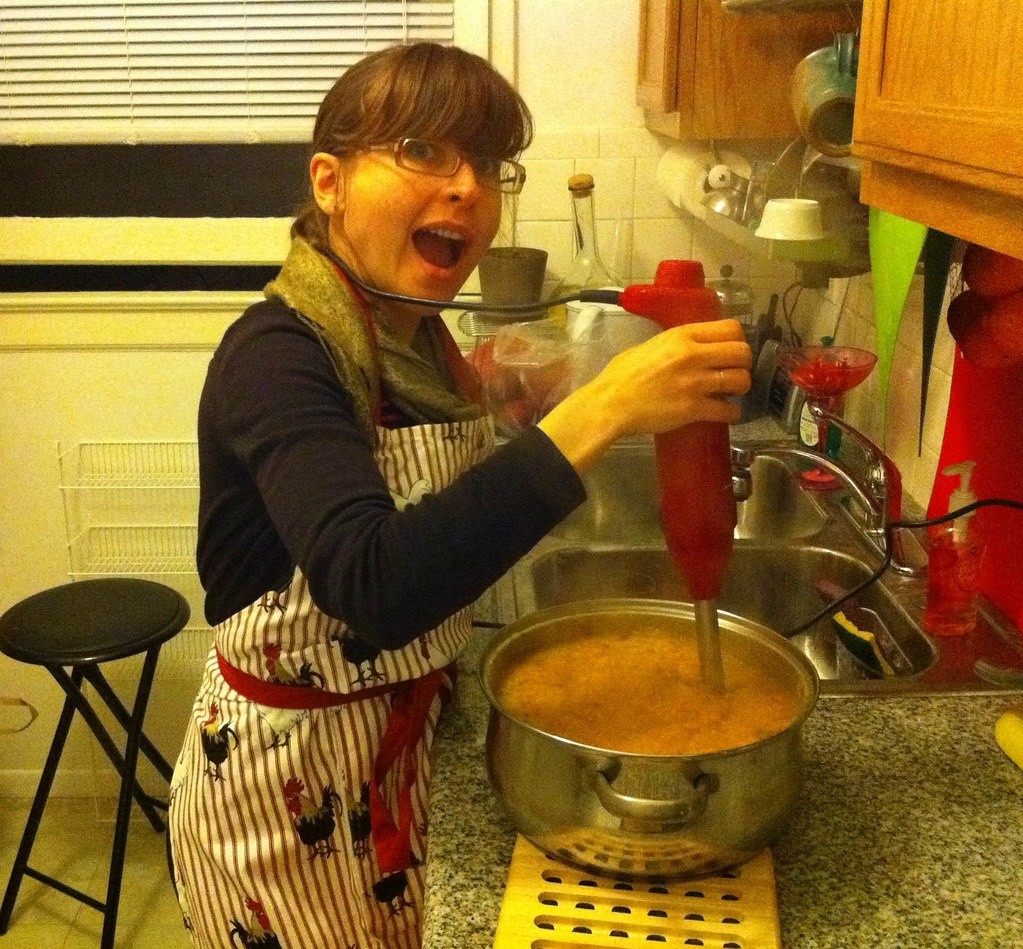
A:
[164, 44, 753, 948]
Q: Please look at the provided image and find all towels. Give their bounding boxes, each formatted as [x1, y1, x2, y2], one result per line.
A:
[994, 704, 1023, 772]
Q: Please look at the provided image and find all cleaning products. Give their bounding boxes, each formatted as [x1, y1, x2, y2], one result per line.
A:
[796, 336, 844, 460]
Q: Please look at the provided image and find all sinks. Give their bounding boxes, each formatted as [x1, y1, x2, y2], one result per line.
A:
[543, 442, 866, 546]
[530, 546, 940, 681]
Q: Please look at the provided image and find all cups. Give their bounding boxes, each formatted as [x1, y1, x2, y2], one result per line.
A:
[786, 30, 860, 158]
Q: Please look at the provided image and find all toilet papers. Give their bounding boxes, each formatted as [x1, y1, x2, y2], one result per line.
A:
[657, 142, 753, 216]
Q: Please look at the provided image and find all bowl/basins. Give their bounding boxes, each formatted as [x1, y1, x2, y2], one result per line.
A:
[479, 247, 548, 325]
[754, 198, 827, 242]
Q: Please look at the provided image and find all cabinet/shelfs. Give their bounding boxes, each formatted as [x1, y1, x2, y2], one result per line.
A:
[635, 0, 1023, 261]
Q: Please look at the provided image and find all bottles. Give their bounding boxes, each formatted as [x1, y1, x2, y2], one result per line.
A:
[546, 173, 630, 303]
[706, 265, 756, 332]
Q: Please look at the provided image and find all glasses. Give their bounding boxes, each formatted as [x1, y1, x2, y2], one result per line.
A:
[360, 138, 526, 194]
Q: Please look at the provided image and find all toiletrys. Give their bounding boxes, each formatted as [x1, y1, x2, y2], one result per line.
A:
[921, 459, 988, 636]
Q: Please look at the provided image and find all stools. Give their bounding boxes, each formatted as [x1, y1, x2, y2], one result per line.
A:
[0, 577, 191, 949]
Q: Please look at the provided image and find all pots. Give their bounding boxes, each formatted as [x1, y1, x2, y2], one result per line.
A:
[474, 597, 821, 882]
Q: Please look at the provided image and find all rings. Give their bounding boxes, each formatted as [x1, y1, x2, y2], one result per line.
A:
[720, 370, 725, 388]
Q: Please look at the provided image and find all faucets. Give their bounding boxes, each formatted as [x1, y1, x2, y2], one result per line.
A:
[729, 404, 904, 536]
